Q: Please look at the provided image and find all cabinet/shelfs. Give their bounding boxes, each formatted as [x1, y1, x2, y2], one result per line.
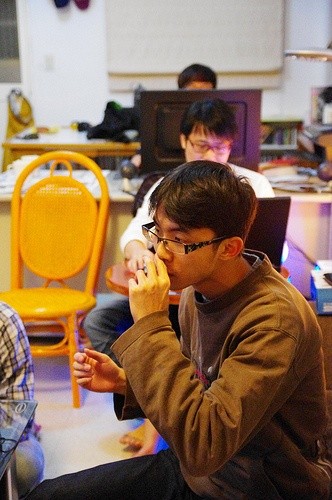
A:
[258, 120, 303, 165]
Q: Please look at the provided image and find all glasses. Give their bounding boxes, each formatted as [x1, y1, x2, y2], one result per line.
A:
[187, 138, 232, 155]
[141, 221, 228, 255]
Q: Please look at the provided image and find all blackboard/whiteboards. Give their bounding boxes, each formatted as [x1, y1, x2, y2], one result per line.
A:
[104, 0, 286, 93]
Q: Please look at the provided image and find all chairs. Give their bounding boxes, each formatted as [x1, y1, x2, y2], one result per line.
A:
[0, 152, 110, 408]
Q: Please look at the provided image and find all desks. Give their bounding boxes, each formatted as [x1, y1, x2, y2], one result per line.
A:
[0, 171, 138, 294]
[271, 175, 331, 299]
[2, 128, 141, 171]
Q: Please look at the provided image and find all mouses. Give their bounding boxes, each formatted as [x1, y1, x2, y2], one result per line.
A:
[137, 255, 152, 274]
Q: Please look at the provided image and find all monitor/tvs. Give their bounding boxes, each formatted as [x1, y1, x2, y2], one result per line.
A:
[139, 89, 262, 174]
[241, 196, 291, 274]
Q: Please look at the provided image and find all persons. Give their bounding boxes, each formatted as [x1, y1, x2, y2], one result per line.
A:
[82, 97, 290, 450]
[26, 160, 332, 500]
[0, 300, 45, 496]
[177, 64, 218, 91]
